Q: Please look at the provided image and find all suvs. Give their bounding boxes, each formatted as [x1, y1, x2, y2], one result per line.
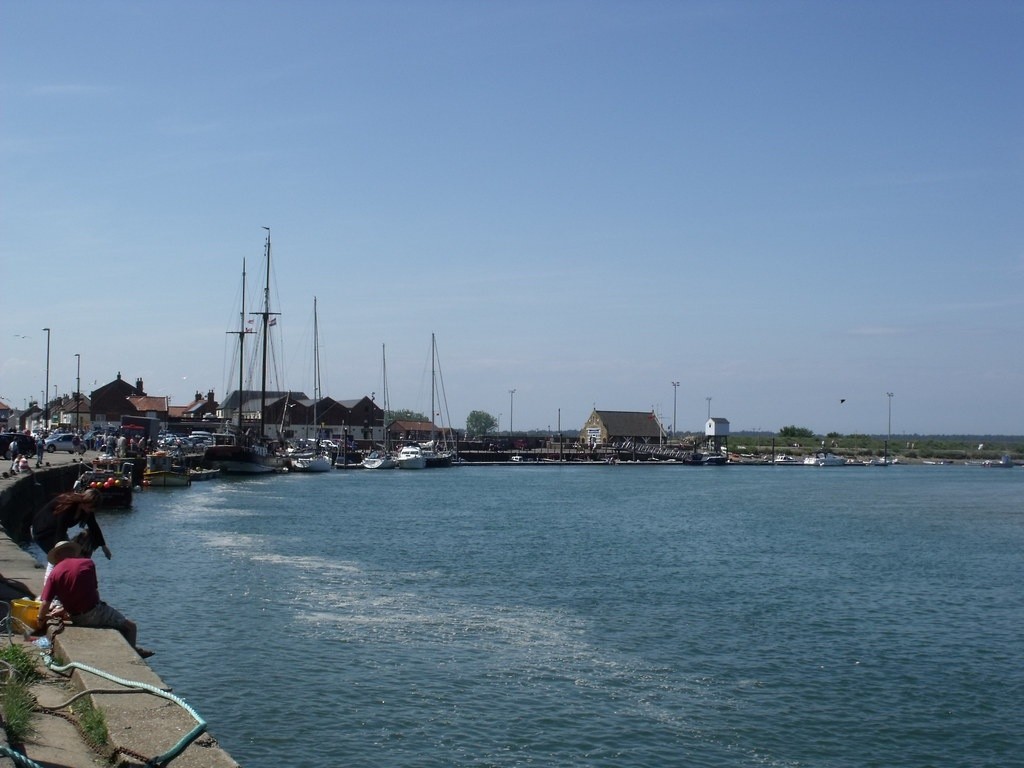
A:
[0, 432, 37, 460]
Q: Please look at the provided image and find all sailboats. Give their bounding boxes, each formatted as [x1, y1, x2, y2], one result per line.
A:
[205, 223, 460, 473]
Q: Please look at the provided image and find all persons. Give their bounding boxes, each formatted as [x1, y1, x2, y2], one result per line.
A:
[574, 440, 621, 453]
[911, 442, 915, 449]
[352, 439, 356, 452]
[19, 453, 42, 486]
[10, 461, 20, 472]
[906, 440, 910, 449]
[42, 528, 100, 604]
[30, 488, 112, 560]
[978, 442, 985, 451]
[830, 438, 834, 448]
[36, 540, 155, 659]
[820, 439, 826, 448]
[835, 442, 838, 448]
[0, 423, 197, 459]
[35, 434, 45, 468]
[315, 439, 321, 454]
[12, 454, 23, 466]
[488, 440, 503, 454]
[8, 435, 19, 471]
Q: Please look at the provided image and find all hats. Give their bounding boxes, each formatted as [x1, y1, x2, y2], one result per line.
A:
[47, 541, 82, 565]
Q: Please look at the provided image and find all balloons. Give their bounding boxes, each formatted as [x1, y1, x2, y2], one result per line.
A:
[108, 477, 115, 485]
[114, 479, 121, 487]
[89, 481, 96, 488]
[141, 479, 148, 486]
[96, 481, 103, 488]
[133, 485, 141, 493]
[103, 481, 110, 489]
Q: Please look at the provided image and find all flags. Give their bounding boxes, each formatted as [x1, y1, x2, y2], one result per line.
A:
[247, 319, 254, 324]
[268, 318, 277, 326]
[246, 327, 252, 333]
[647, 415, 655, 422]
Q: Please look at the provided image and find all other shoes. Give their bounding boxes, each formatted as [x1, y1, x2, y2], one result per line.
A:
[135, 647, 154, 660]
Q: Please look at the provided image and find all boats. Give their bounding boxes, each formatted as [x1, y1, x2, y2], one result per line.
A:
[190, 466, 220, 481]
[141, 451, 192, 486]
[983, 456, 1014, 468]
[73, 463, 133, 511]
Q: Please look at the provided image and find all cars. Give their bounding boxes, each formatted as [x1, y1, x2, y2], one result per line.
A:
[42, 433, 87, 454]
[82, 429, 117, 450]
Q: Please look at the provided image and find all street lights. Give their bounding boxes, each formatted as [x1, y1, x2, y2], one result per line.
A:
[41, 391, 44, 403]
[54, 384, 57, 399]
[42, 328, 50, 432]
[75, 354, 80, 432]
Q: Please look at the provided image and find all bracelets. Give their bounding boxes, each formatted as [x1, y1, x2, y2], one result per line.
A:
[36, 615, 45, 622]
